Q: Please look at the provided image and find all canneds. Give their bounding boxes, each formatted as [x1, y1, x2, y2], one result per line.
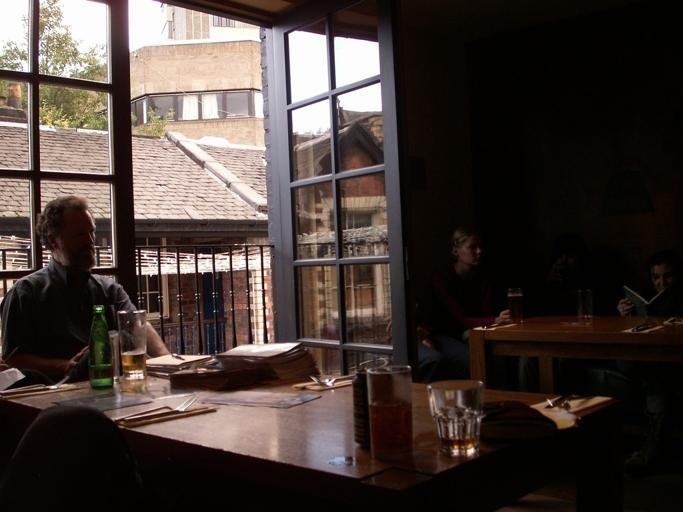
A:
[350, 369, 394, 452]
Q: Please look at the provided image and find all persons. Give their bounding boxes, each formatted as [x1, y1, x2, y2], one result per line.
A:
[595, 251, 682, 477]
[0, 196, 172, 381]
[0, 406, 152, 510]
[429, 223, 512, 339]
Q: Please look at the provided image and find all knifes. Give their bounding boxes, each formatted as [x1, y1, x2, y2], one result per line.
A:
[296, 375, 350, 390]
[126, 403, 215, 421]
[544, 395, 600, 410]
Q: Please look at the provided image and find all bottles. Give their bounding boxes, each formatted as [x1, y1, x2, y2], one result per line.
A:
[87, 304, 114, 387]
[351, 368, 370, 448]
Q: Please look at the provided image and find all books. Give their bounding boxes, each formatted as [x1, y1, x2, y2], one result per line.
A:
[621, 285, 673, 316]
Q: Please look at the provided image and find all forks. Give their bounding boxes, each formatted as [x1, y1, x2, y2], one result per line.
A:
[309, 375, 356, 390]
[1, 376, 69, 394]
[114, 393, 198, 423]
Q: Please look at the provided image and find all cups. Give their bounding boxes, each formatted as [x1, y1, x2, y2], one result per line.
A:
[368, 363, 417, 458]
[576, 287, 592, 327]
[426, 377, 483, 460]
[107, 330, 121, 380]
[507, 287, 524, 325]
[116, 309, 149, 380]
